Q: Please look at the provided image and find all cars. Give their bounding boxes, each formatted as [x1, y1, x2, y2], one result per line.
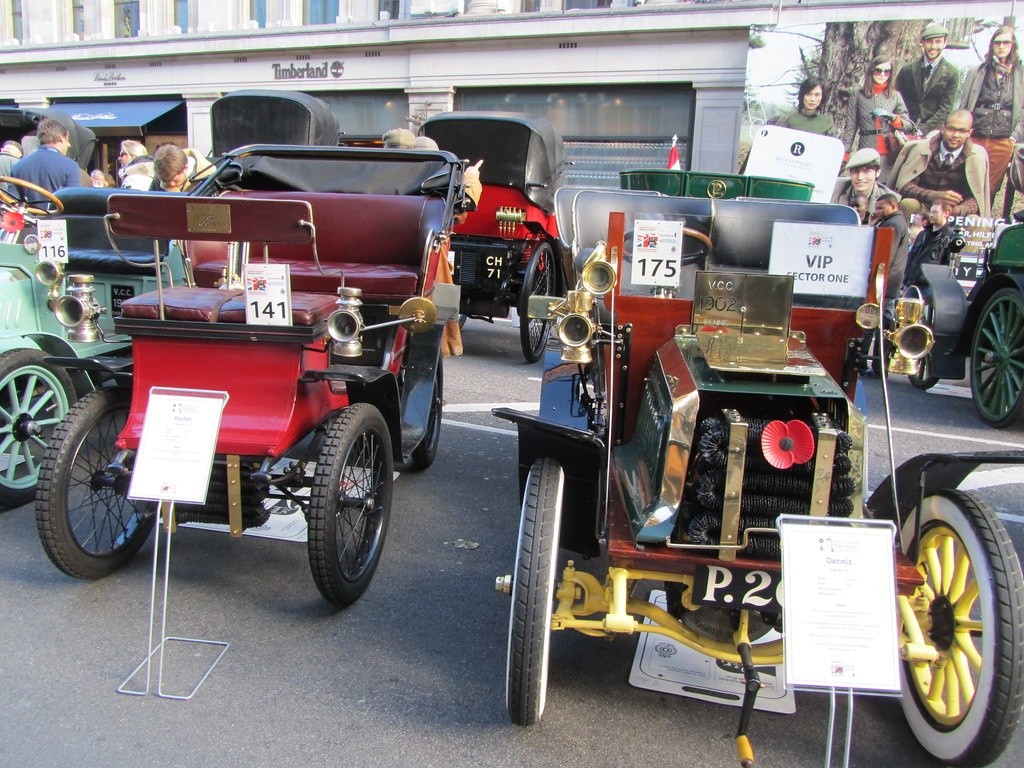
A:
[862, 223, 1023, 429]
[0, 92, 341, 515]
[414, 111, 567, 364]
[494, 164, 1024, 767]
[35, 141, 472, 611]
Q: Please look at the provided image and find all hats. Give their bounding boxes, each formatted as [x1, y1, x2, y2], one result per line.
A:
[1, 140, 23, 158]
[382, 128, 415, 146]
[845, 148, 880, 168]
[410, 136, 439, 151]
[921, 24, 949, 39]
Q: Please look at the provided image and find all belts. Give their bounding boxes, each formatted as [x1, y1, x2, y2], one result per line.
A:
[858, 129, 893, 136]
[975, 102, 1013, 112]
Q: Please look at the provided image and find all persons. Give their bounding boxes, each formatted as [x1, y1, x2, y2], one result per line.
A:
[381, 129, 484, 257]
[849, 194, 956, 378]
[0, 118, 219, 211]
[775, 24, 1024, 253]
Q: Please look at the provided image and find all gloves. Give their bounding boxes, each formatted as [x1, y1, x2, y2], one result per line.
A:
[870, 108, 894, 121]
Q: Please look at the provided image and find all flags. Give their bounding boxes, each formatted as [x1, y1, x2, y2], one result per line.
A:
[667, 135, 681, 171]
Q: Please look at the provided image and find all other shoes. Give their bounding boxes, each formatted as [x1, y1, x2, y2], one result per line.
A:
[865, 368, 888, 380]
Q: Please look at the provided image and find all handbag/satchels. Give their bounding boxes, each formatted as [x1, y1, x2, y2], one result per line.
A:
[887, 119, 926, 162]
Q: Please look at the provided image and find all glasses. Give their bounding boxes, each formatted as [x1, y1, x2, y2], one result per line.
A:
[118, 152, 130, 158]
[871, 67, 892, 77]
[992, 41, 1013, 47]
[943, 123, 971, 135]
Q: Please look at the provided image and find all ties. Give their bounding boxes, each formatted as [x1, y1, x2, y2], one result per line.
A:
[941, 153, 954, 170]
[925, 65, 933, 79]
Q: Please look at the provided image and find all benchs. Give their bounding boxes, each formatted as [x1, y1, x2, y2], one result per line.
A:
[44, 186, 170, 279]
[554, 185, 663, 291]
[99, 192, 337, 324]
[184, 189, 445, 306]
[575, 186, 862, 313]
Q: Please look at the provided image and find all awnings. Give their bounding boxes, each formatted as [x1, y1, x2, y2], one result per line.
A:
[0, 100, 186, 136]
